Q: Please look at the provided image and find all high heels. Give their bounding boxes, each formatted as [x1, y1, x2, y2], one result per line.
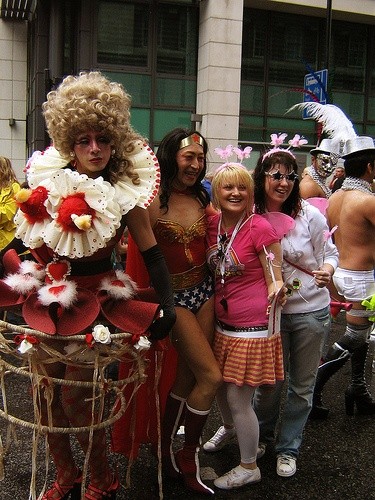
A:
[41, 465, 84, 500]
[83, 467, 121, 500]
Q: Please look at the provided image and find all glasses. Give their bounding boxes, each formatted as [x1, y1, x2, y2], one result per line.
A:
[264, 169, 300, 182]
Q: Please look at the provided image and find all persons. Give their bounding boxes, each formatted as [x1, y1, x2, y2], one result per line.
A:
[0, 155, 23, 279]
[312, 137, 375, 411]
[0, 72, 178, 500]
[252, 134, 339, 477]
[111, 129, 223, 494]
[205, 146, 291, 488]
[298, 139, 341, 201]
[328, 167, 345, 194]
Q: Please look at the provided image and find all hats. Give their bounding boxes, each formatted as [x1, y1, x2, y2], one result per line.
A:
[285, 102, 375, 158]
[309, 138, 333, 157]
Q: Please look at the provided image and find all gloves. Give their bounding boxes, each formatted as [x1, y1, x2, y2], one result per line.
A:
[142, 243, 177, 340]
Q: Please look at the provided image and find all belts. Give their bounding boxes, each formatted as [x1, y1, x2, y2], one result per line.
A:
[170, 259, 211, 291]
[214, 317, 269, 333]
[46, 256, 113, 277]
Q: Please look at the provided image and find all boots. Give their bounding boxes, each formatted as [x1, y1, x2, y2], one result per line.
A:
[309, 334, 351, 419]
[150, 390, 188, 481]
[173, 400, 215, 499]
[344, 321, 375, 419]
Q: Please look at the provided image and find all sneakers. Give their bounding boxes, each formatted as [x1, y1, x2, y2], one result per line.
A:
[213, 464, 262, 489]
[276, 454, 296, 477]
[256, 442, 267, 459]
[203, 425, 237, 452]
